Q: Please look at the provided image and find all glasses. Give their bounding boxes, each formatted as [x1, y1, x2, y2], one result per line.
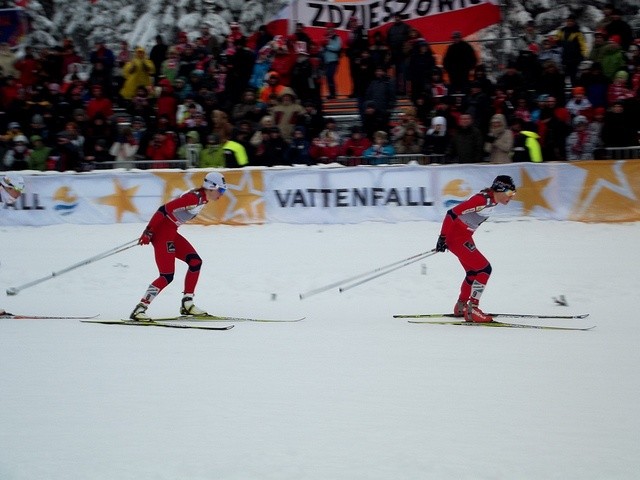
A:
[216, 187, 226, 194]
[503, 188, 517, 197]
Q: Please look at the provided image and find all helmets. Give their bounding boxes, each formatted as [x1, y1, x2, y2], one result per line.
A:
[1, 173, 25, 194]
[202, 172, 228, 191]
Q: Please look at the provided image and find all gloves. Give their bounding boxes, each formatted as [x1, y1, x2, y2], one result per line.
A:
[436, 235, 446, 253]
[138, 230, 154, 246]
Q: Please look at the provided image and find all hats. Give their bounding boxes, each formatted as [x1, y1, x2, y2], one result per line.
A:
[492, 175, 516, 191]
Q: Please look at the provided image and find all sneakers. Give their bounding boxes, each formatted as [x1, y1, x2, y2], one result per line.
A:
[454, 301, 468, 315]
[464, 304, 492, 323]
[179, 294, 204, 314]
[130, 303, 152, 320]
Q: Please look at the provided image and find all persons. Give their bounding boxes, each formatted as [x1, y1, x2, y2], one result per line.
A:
[483, 113, 516, 163]
[129, 171, 229, 322]
[424, 115, 458, 162]
[341, 126, 372, 164]
[306, 116, 346, 163]
[395, 120, 424, 155]
[364, 130, 395, 164]
[350, 9, 635, 119]
[458, 114, 482, 163]
[437, 175, 517, 325]
[323, 24, 346, 99]
[564, 114, 598, 160]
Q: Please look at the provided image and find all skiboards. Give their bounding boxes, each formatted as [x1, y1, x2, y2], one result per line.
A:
[80, 313, 304, 330]
[394, 315, 596, 330]
[0, 314, 100, 320]
